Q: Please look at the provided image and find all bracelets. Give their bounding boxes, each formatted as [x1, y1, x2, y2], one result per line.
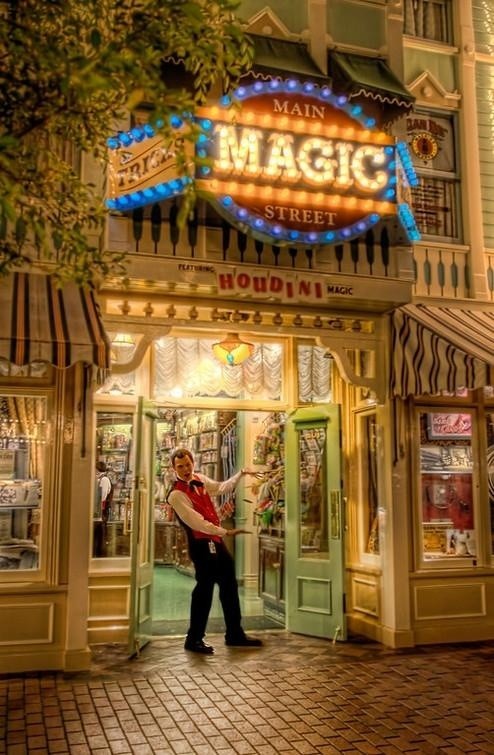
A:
[243, 468, 244, 474]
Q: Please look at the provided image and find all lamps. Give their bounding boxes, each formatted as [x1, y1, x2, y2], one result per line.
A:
[210, 332, 256, 368]
[111, 332, 135, 348]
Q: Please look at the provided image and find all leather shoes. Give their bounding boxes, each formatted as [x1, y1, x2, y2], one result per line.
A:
[184, 636, 212, 653]
[225, 637, 261, 647]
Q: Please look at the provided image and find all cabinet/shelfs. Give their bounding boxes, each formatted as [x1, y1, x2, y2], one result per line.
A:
[149, 615, 284, 636]
[254, 535, 285, 626]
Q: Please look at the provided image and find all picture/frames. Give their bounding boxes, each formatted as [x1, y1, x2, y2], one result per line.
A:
[426, 412, 472, 441]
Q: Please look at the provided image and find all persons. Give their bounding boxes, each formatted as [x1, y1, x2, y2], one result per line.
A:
[93, 461, 113, 558]
[166, 448, 263, 654]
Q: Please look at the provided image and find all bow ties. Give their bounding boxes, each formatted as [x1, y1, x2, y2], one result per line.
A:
[189, 480, 204, 491]
